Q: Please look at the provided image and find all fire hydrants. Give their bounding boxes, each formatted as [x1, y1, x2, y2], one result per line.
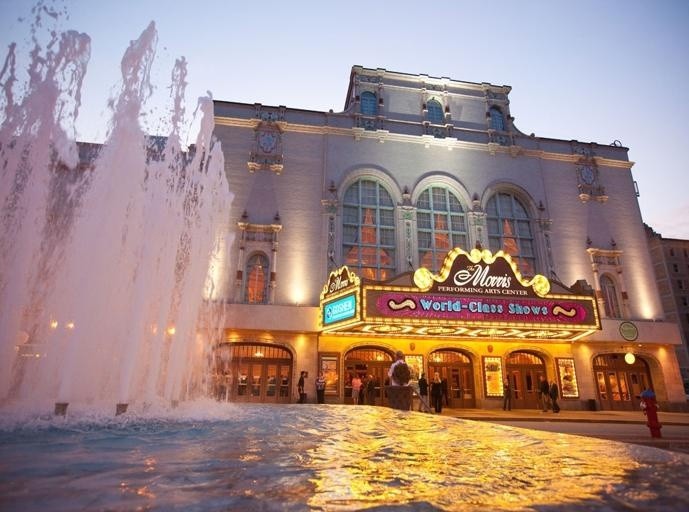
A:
[640, 387, 663, 438]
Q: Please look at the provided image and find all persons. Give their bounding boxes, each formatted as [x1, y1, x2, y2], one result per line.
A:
[351, 373, 376, 406]
[418, 373, 429, 412]
[315, 371, 326, 403]
[549, 379, 560, 413]
[539, 375, 550, 412]
[387, 350, 412, 386]
[502, 378, 512, 411]
[298, 371, 306, 403]
[430, 372, 448, 414]
[216, 366, 288, 396]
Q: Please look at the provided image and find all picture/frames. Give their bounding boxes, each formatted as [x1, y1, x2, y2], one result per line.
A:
[555, 357, 581, 399]
[405, 354, 424, 398]
[482, 356, 505, 398]
